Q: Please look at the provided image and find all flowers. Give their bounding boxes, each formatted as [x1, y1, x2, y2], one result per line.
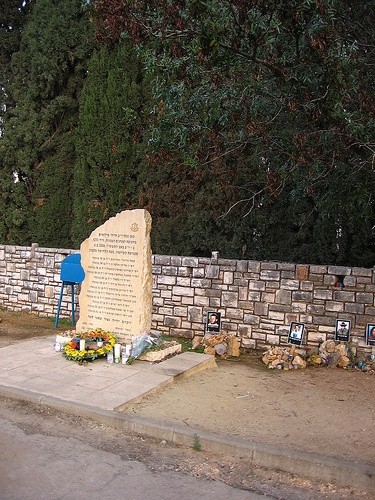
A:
[60, 327, 116, 365]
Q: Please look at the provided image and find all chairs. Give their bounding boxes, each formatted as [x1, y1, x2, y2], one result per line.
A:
[54, 254, 85, 329]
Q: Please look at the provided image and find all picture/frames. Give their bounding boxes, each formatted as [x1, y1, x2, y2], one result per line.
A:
[205, 311, 221, 334]
[286, 321, 305, 347]
[365, 323, 375, 346]
[334, 319, 350, 342]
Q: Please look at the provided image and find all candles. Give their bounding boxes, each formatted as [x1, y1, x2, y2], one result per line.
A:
[114, 344, 120, 363]
[121, 347, 128, 364]
[132, 336, 137, 354]
[79, 339, 86, 352]
[126, 344, 132, 357]
[106, 348, 114, 365]
[97, 342, 103, 348]
